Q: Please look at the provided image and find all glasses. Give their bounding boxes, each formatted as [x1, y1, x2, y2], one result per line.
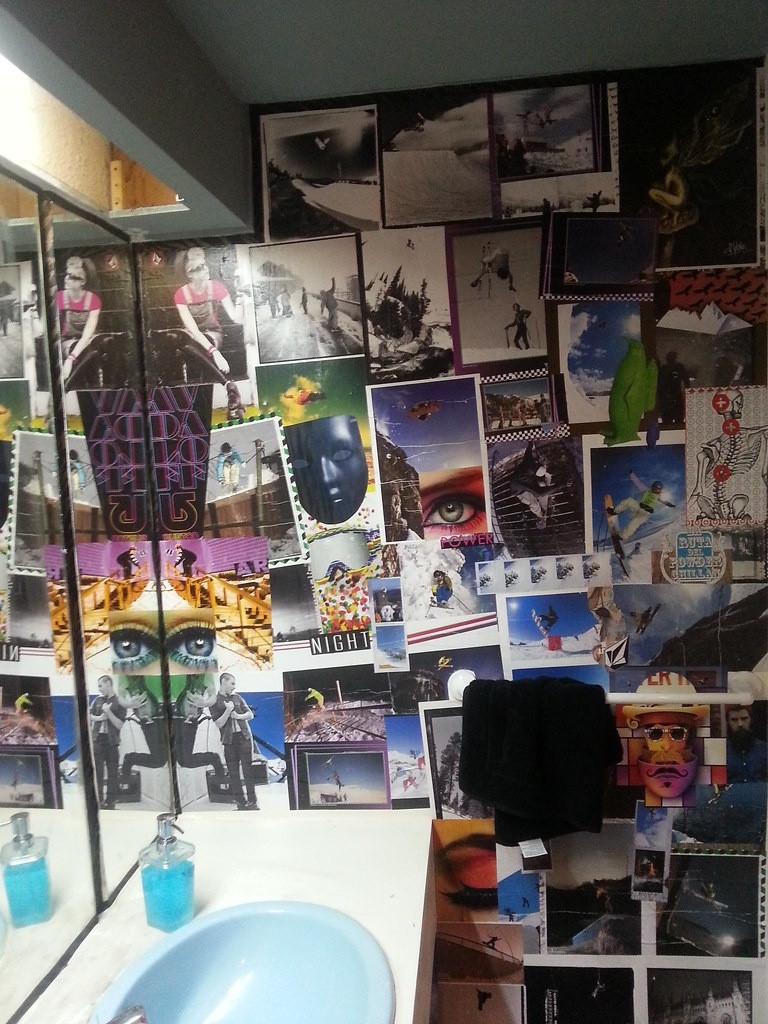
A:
[644, 725, 691, 742]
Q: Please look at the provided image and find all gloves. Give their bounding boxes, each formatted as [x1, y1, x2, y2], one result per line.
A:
[629, 468, 632, 473]
[672, 504, 676, 507]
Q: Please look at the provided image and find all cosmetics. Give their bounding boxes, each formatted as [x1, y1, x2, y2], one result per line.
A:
[1, 812, 54, 928]
[138, 812, 198, 934]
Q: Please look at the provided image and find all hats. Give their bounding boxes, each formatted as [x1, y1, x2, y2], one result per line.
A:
[620, 672, 712, 722]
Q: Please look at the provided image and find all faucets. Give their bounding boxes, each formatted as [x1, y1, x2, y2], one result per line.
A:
[105, 1002, 149, 1023]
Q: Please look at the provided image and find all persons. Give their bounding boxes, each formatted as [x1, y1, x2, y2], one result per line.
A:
[304, 688, 499, 923]
[377, 464, 488, 621]
[726, 705, 768, 784]
[607, 469, 677, 540]
[52, 449, 87, 492]
[660, 351, 691, 424]
[268, 277, 341, 406]
[56, 256, 117, 394]
[88, 542, 262, 811]
[166, 248, 244, 375]
[216, 442, 247, 493]
[486, 394, 575, 529]
[538, 605, 559, 632]
[0, 282, 15, 339]
[472, 247, 531, 350]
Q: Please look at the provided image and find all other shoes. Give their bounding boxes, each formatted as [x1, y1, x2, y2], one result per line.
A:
[611, 531, 623, 541]
[605, 505, 617, 517]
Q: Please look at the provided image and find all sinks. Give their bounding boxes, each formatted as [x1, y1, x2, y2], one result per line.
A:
[94, 898, 402, 1024]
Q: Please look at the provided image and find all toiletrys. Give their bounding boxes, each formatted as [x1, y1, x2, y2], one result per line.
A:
[2, 812, 55, 930]
[133, 810, 197, 933]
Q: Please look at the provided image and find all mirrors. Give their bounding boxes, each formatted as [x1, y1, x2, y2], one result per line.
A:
[41, 190, 180, 910]
[0, 166, 99, 1024]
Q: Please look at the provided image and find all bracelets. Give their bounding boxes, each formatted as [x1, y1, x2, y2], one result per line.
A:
[68, 354, 77, 362]
[207, 345, 217, 355]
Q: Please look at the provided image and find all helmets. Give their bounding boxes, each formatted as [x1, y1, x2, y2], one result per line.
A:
[652, 480, 665, 490]
